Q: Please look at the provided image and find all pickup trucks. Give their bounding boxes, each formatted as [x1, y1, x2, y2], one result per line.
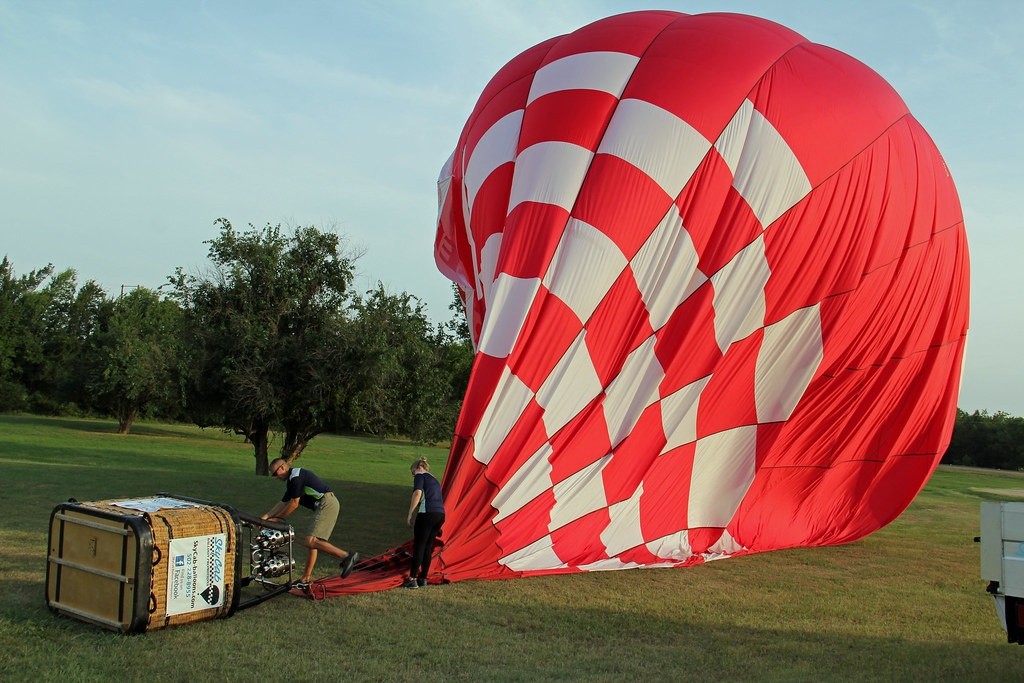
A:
[972, 498, 1024, 648]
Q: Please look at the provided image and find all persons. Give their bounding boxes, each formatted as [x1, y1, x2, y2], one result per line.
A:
[257, 458, 358, 589]
[401, 456, 445, 589]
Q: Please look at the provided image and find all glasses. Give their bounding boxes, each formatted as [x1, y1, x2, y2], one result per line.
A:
[271, 462, 284, 476]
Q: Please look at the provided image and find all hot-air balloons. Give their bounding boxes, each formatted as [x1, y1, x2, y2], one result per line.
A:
[42, 9, 972, 636]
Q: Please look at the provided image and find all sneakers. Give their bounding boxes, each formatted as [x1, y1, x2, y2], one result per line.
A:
[339, 552, 359, 579]
[291, 580, 312, 590]
[417, 578, 427, 587]
[399, 577, 419, 589]
